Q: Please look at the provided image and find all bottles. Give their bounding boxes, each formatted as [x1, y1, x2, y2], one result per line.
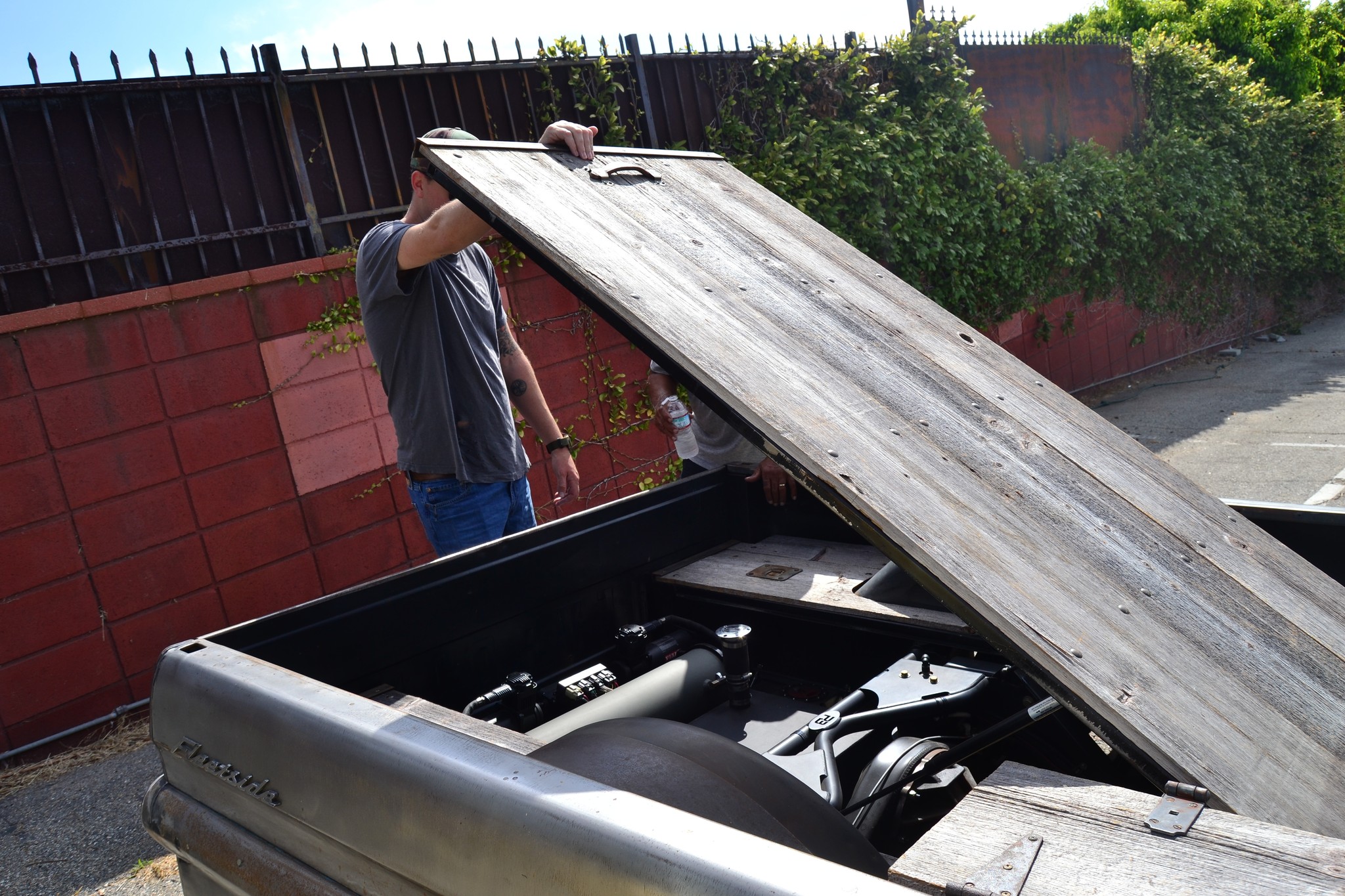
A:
[666, 394, 699, 459]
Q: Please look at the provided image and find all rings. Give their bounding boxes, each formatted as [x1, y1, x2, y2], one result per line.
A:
[779, 484, 785, 486]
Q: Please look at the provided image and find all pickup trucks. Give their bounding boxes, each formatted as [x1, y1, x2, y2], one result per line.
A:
[137, 449, 1345, 895]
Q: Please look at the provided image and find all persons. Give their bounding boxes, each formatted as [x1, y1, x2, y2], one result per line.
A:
[355, 120, 600, 559]
[646, 359, 797, 508]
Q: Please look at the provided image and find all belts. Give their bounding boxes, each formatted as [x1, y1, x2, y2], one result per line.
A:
[401, 468, 459, 482]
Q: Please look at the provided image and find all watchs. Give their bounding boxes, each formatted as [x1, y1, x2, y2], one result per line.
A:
[546, 434, 572, 455]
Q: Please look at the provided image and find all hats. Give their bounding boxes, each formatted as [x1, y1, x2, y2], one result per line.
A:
[411, 125, 483, 173]
